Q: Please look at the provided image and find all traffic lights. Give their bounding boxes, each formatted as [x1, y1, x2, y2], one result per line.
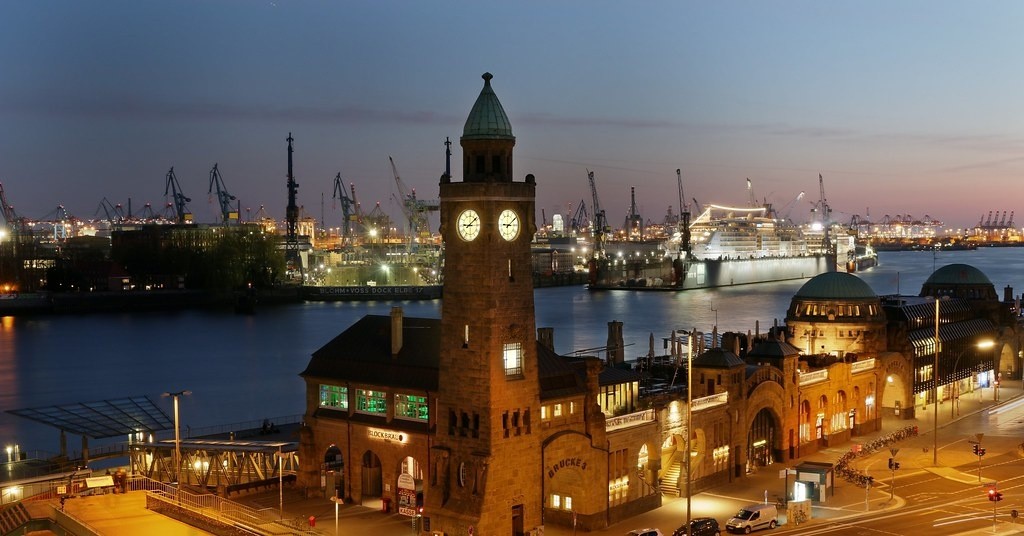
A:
[973, 444, 986, 456]
[988, 482, 1002, 501]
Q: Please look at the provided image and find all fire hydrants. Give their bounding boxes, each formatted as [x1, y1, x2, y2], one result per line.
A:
[380, 498, 391, 514]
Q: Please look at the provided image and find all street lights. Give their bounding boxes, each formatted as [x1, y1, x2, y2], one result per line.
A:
[925, 296, 951, 463]
[676, 328, 703, 536]
[1019, 350, 1024, 392]
[161, 391, 193, 520]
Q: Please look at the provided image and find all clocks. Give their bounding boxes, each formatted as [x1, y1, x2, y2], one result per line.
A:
[498, 210, 519, 241]
[457, 208, 480, 242]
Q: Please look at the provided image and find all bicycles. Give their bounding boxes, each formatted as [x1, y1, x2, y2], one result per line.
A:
[833, 423, 918, 490]
[792, 509, 807, 526]
[774, 494, 792, 510]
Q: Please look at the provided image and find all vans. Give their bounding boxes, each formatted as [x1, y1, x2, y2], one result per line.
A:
[726, 504, 778, 534]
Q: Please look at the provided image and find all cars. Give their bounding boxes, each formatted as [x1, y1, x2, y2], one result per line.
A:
[673, 517, 721, 536]
[626, 528, 663, 536]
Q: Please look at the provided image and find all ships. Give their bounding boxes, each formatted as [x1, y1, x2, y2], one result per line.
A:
[0, 128, 1024, 315]
